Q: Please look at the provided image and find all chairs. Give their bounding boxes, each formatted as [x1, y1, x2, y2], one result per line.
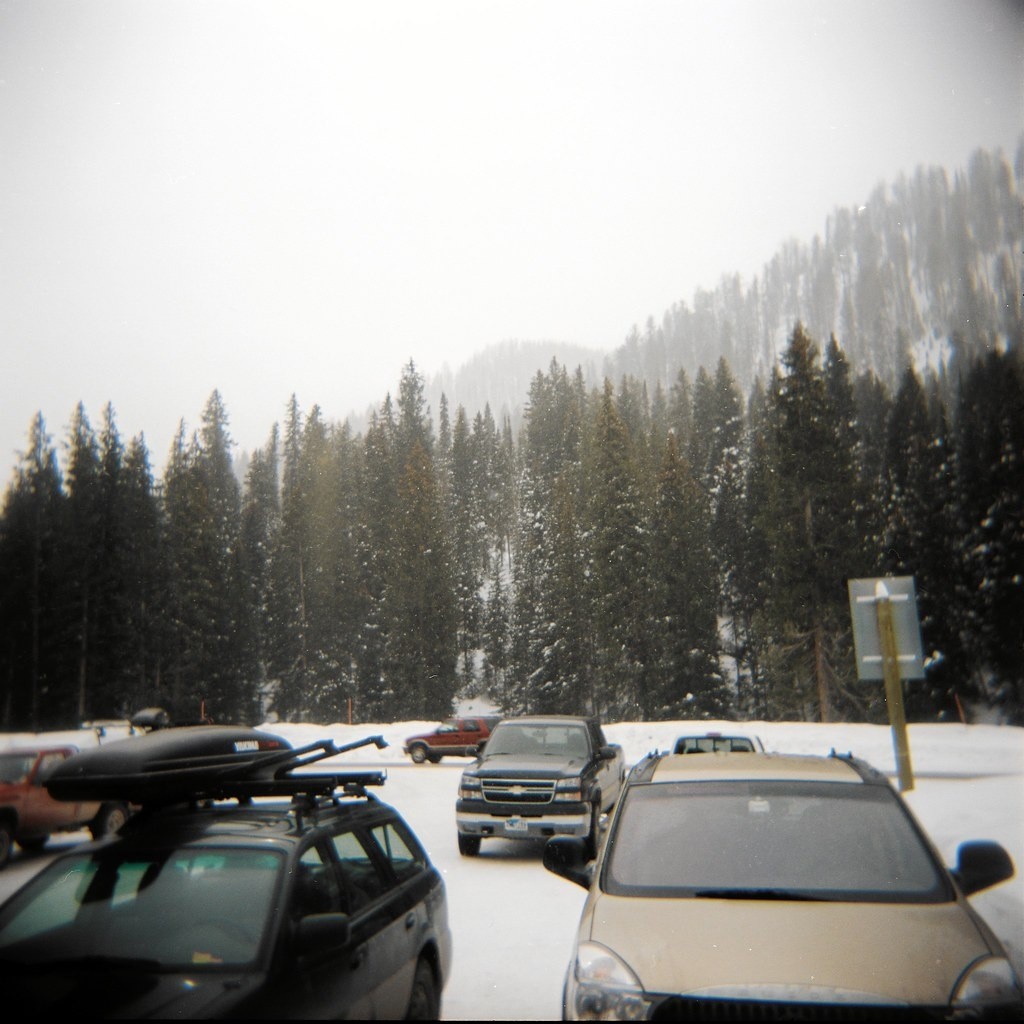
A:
[134, 868, 204, 930]
[730, 745, 749, 752]
[686, 747, 705, 754]
[561, 734, 586, 756]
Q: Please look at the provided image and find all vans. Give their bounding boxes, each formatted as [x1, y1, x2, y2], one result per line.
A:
[675, 730, 770, 760]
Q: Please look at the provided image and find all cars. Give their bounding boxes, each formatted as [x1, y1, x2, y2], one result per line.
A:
[544, 746, 1024, 1024]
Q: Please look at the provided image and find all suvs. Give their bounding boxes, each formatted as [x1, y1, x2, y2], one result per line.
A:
[402, 715, 501, 765]
[0, 749, 134, 868]
[0, 708, 459, 1024]
[455, 714, 627, 856]
